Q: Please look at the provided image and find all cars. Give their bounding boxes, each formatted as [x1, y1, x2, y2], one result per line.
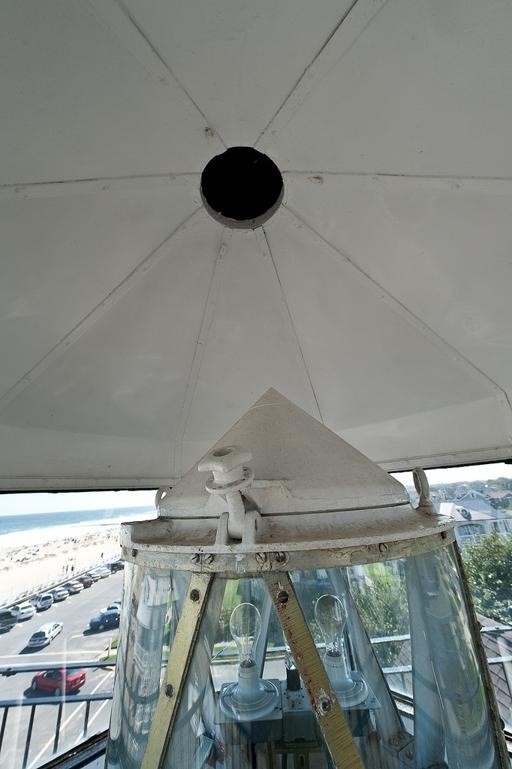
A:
[31, 668, 86, 696]
[0, 557, 124, 650]
